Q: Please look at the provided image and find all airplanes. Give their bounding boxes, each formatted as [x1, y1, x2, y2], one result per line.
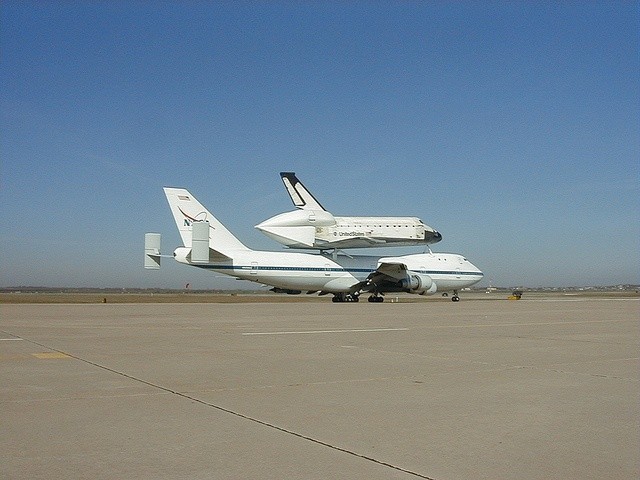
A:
[142, 171, 485, 304]
